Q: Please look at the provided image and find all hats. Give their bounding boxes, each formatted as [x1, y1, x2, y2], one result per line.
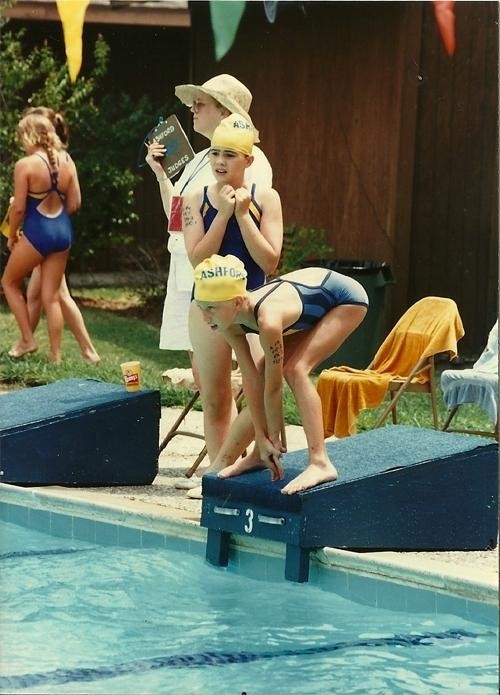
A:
[174, 74, 260, 144]
[210, 113, 253, 156]
[194, 255, 248, 302]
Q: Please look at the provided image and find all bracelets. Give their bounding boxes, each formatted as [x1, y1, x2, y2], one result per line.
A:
[157, 175, 168, 182]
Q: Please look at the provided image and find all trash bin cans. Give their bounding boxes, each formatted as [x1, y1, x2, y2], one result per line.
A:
[302, 258, 391, 376]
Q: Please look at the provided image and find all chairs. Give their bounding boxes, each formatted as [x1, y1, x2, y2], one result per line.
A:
[440, 317, 498, 445]
[153, 334, 288, 477]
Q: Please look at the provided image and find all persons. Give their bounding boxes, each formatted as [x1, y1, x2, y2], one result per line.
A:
[181, 112, 285, 475]
[9, 105, 102, 366]
[144, 72, 278, 501]
[170, 253, 371, 498]
[0, 113, 82, 364]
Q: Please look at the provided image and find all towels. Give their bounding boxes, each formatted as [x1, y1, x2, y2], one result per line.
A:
[441, 319, 498, 426]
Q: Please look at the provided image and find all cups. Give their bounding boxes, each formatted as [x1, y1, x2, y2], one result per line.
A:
[119, 360, 141, 392]
[167, 195, 186, 235]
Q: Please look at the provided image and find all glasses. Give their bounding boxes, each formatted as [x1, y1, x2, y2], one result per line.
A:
[192, 100, 208, 113]
